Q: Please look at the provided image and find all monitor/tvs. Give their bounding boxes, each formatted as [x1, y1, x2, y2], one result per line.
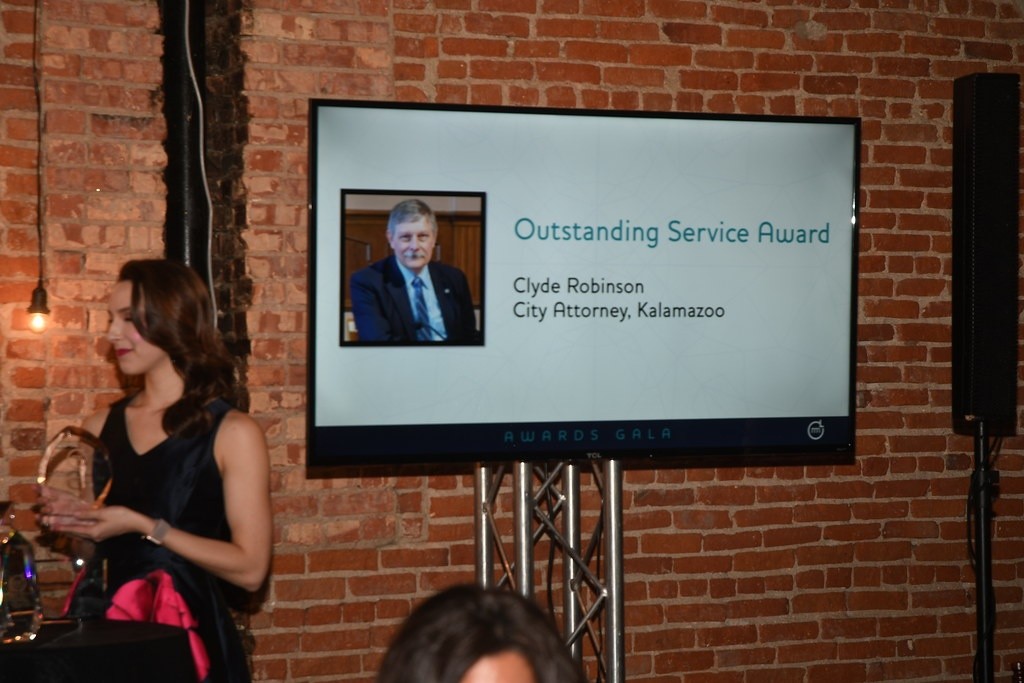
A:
[305, 97, 863, 467]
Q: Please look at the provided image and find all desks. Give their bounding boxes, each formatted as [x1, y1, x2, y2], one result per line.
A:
[0, 617, 201, 683]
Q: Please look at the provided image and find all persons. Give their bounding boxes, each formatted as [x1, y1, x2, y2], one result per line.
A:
[378, 584, 587, 683]
[36, 258, 270, 683]
[348, 199, 477, 343]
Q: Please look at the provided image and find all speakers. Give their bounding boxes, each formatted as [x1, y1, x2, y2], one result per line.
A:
[952, 72, 1022, 438]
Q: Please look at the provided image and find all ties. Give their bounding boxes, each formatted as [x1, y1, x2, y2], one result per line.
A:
[411, 278, 431, 341]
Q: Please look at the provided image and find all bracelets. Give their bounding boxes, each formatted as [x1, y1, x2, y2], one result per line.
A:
[140, 518, 171, 545]
